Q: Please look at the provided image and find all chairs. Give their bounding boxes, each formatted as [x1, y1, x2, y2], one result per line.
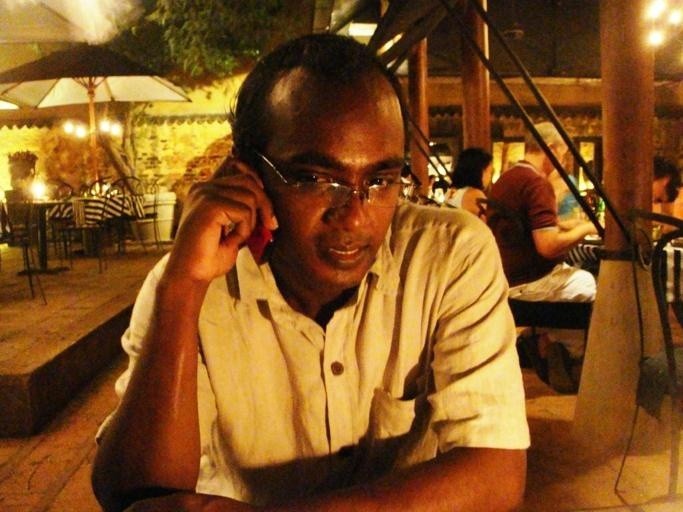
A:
[417, 192, 592, 347]
[0, 176, 165, 305]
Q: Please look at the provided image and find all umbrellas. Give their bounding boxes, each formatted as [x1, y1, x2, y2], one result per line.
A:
[0, 37, 195, 191]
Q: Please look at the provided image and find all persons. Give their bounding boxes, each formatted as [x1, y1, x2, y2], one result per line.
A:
[91, 33, 532, 511]
[402, 119, 682, 396]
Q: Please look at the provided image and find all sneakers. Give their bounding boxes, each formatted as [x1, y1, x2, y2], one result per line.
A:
[547, 340, 577, 393]
[519, 333, 549, 384]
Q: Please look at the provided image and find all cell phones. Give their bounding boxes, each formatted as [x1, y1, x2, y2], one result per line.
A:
[246, 215, 273, 267]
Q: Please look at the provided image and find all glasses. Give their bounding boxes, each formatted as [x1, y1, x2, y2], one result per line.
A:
[254, 151, 416, 210]
